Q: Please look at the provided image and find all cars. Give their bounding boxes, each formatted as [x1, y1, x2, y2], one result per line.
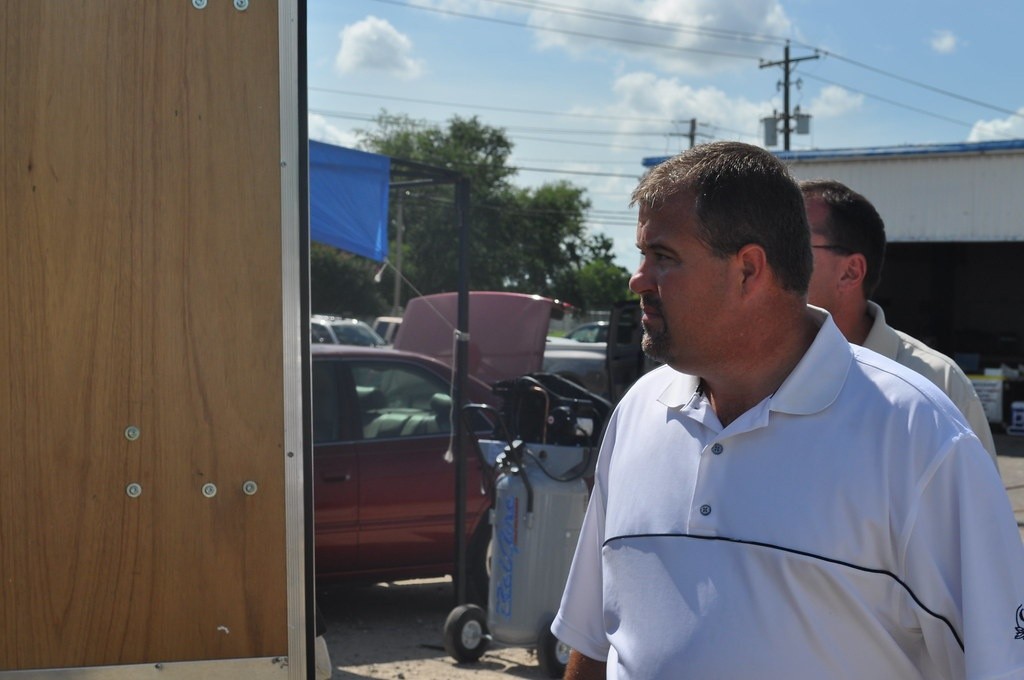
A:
[310, 314, 395, 349]
[310, 343, 502, 597]
[373, 316, 403, 345]
[541, 320, 611, 398]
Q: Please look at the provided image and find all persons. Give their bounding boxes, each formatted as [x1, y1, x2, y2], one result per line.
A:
[550, 142, 1024, 678]
[796, 177, 1001, 476]
